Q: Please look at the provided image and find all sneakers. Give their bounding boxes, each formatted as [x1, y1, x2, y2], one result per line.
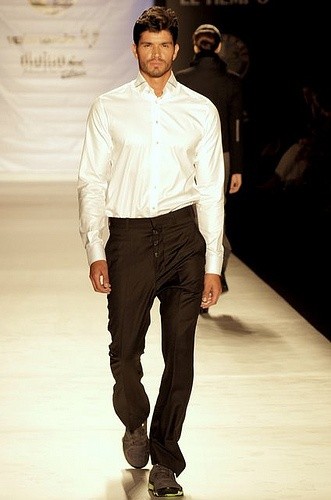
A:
[146, 464, 185, 498]
[122, 419, 149, 468]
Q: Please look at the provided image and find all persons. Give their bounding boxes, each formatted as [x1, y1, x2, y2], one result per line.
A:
[173, 25, 246, 313]
[255, 82, 331, 213]
[76, 6, 225, 498]
